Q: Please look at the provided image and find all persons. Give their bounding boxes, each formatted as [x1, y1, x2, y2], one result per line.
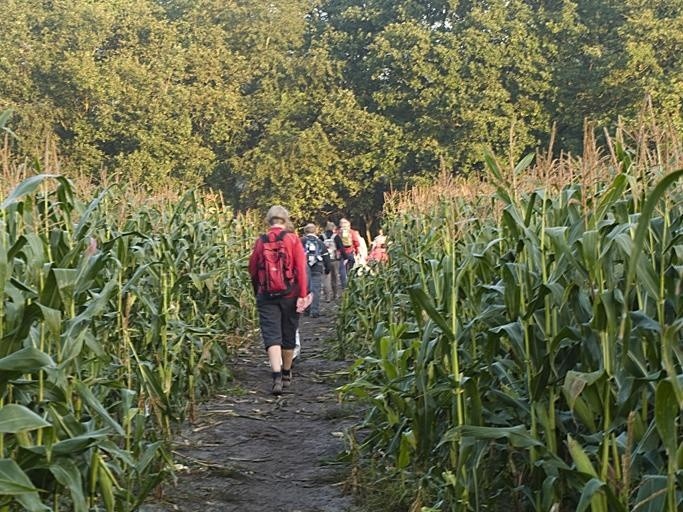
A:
[247, 204, 306, 391]
[365, 228, 393, 264]
[318, 221, 348, 303]
[262, 222, 313, 365]
[335, 218, 360, 291]
[297, 223, 331, 318]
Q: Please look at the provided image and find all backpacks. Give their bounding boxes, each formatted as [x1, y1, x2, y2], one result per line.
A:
[340, 229, 353, 248]
[303, 236, 321, 268]
[320, 233, 339, 260]
[256, 230, 296, 299]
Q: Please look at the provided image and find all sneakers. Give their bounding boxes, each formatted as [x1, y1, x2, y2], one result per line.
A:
[272, 376, 283, 395]
[282, 371, 292, 387]
[304, 294, 342, 318]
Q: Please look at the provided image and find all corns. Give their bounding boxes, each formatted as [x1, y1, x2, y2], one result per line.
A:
[565, 434, 597, 477]
